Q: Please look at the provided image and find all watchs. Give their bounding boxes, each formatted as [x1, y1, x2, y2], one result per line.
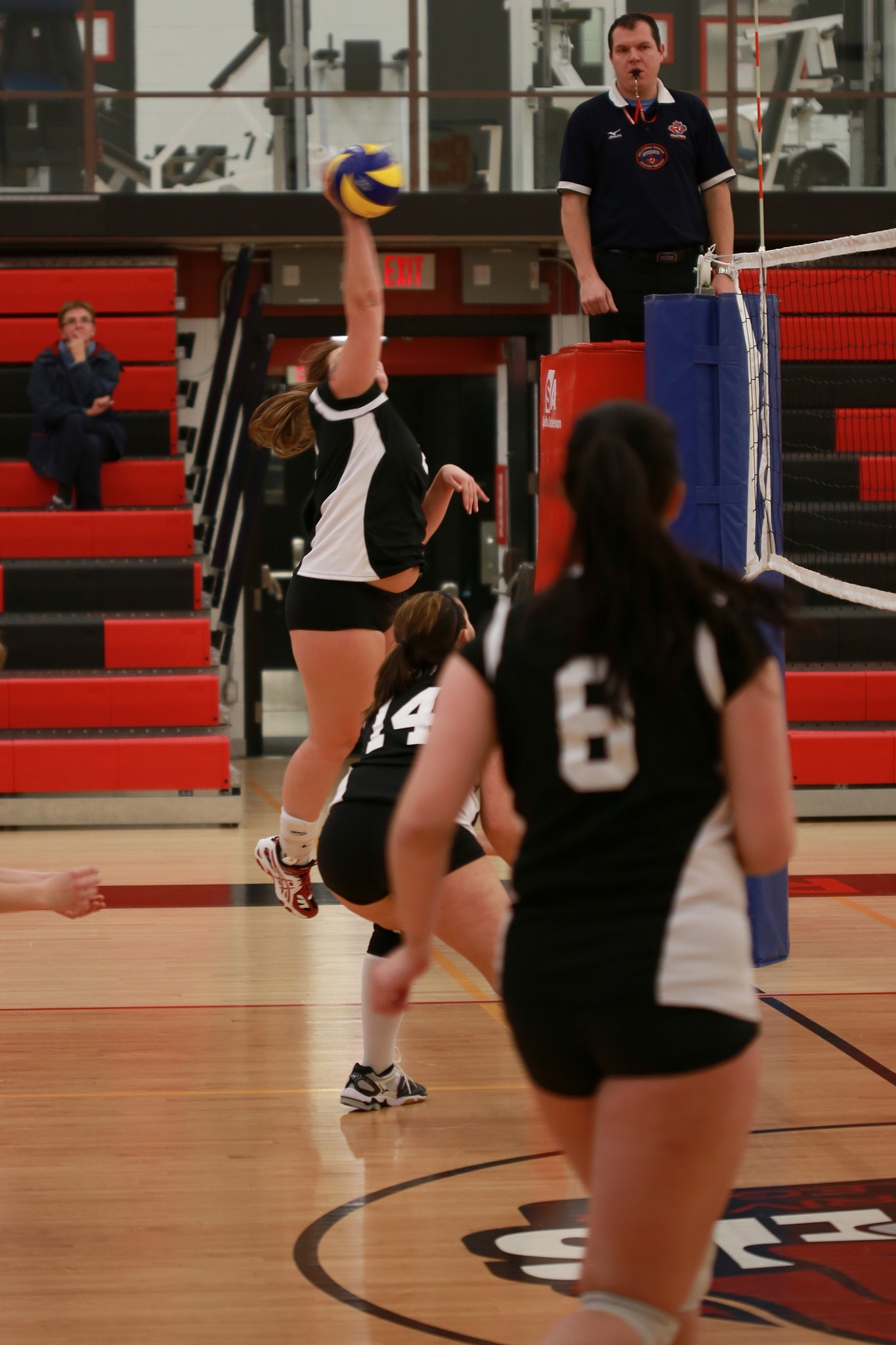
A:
[716, 266, 737, 281]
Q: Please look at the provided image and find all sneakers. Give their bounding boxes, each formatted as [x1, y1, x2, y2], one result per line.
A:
[338, 1046, 427, 1111]
[253, 834, 321, 920]
[339, 1108, 425, 1159]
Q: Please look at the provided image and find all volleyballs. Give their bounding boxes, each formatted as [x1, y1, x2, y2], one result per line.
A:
[325, 143, 403, 218]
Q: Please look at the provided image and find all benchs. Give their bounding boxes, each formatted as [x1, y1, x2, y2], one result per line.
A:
[0, 266, 232, 795]
[735, 267, 896, 786]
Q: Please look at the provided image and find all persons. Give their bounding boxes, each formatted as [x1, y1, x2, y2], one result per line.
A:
[24, 299, 119, 510]
[0, 867, 105, 919]
[366, 394, 796, 1345]
[246, 170, 493, 922]
[318, 590, 524, 1113]
[554, 14, 739, 344]
